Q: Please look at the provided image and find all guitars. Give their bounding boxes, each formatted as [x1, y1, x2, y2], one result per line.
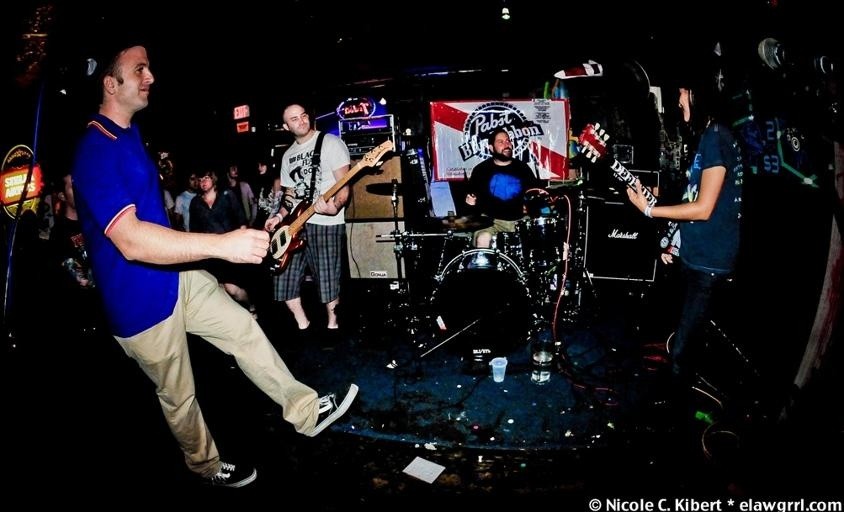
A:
[579, 122, 681, 266]
[261, 140, 393, 275]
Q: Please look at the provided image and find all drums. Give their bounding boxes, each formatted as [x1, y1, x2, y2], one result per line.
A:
[518, 217, 566, 267]
[430, 248, 532, 361]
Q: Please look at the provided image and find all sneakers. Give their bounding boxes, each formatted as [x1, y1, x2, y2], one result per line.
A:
[206, 459, 259, 489]
[311, 382, 359, 439]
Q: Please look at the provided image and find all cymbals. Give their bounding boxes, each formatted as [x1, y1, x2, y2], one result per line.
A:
[423, 216, 495, 233]
[365, 182, 417, 197]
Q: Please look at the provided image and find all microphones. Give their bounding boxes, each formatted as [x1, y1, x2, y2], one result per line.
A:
[391, 178, 397, 202]
[86, 58, 97, 76]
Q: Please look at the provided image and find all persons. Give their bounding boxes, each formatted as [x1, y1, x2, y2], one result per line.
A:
[602, 70, 738, 406]
[174, 172, 199, 233]
[465, 130, 539, 250]
[72, 46, 360, 489]
[49, 173, 93, 290]
[256, 160, 281, 231]
[224, 163, 257, 227]
[190, 165, 258, 321]
[265, 104, 350, 346]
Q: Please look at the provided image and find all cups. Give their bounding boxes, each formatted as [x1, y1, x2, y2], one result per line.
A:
[491, 358, 508, 383]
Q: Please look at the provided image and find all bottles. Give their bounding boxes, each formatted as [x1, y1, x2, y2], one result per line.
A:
[532, 331, 553, 385]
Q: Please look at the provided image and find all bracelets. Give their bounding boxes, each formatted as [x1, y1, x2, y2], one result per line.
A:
[276, 213, 283, 222]
[644, 206, 653, 219]
